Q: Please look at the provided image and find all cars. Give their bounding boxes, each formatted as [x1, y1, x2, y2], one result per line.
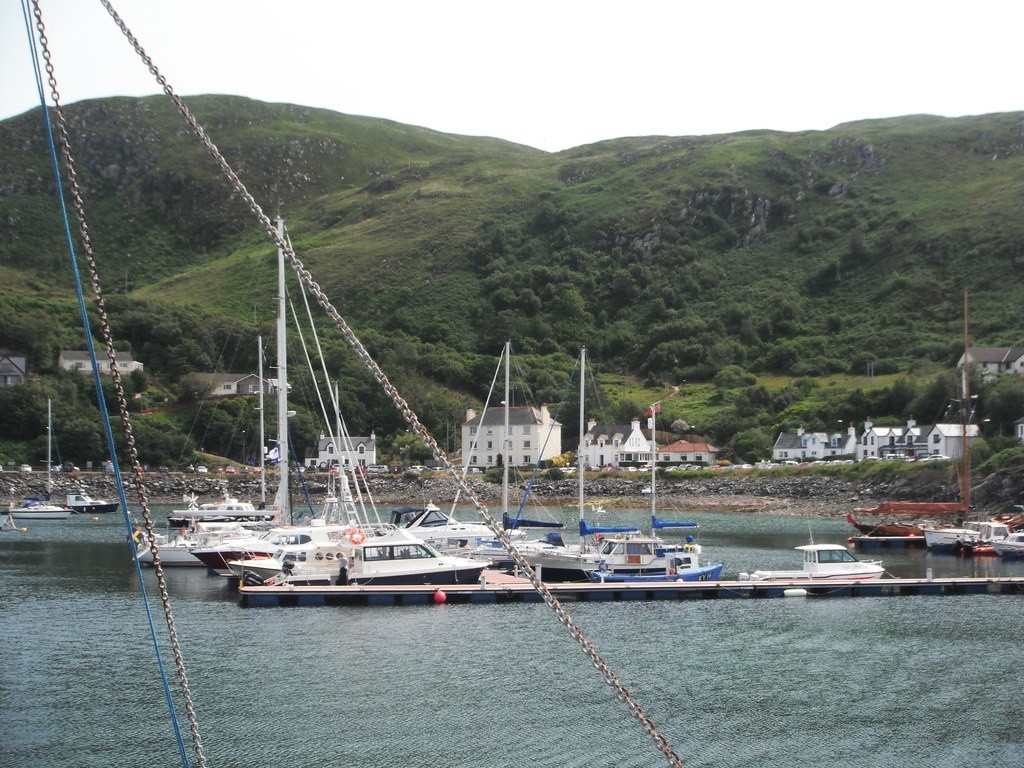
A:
[18, 464, 32, 473]
[754, 453, 951, 469]
[732, 463, 753, 468]
[157, 465, 169, 473]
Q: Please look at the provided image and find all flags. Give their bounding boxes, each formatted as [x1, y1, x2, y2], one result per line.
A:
[127, 526, 143, 548]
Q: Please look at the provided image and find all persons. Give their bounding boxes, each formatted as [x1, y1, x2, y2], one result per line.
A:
[333, 552, 349, 586]
[145, 464, 148, 472]
[953, 535, 965, 553]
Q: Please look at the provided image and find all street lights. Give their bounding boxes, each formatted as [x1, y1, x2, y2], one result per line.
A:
[242, 430, 246, 467]
[405, 430, 410, 469]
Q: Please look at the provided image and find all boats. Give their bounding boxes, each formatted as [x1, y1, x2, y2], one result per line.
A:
[60, 492, 121, 514]
[960, 543, 995, 554]
[989, 530, 1024, 562]
[922, 518, 1016, 548]
[737, 515, 888, 581]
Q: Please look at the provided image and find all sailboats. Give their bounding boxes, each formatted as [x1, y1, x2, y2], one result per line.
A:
[136, 212, 733, 589]
[842, 288, 1024, 538]
[9, 398, 74, 520]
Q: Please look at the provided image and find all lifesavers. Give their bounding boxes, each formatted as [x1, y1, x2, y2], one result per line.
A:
[349, 528, 366, 545]
[319, 461, 328, 470]
